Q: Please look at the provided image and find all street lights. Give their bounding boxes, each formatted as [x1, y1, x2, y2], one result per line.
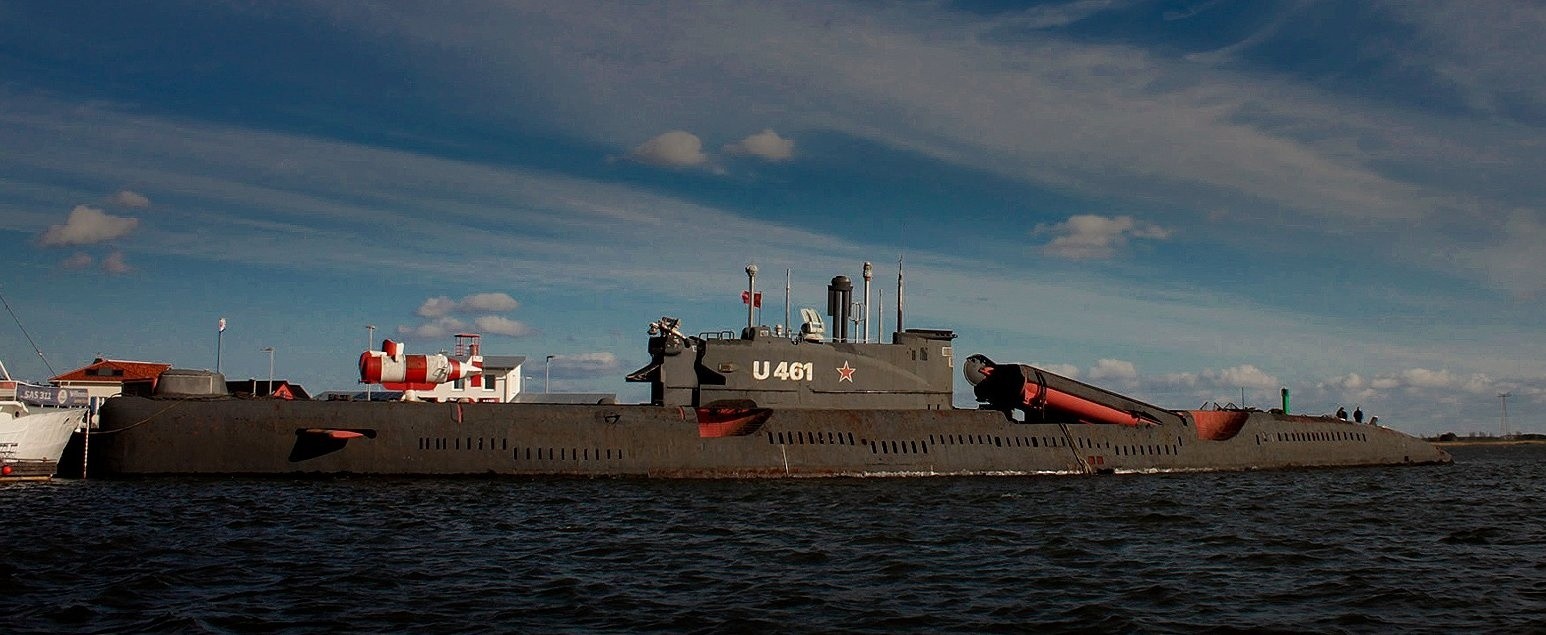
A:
[259, 346, 275, 396]
[365, 324, 376, 402]
[545, 355, 555, 394]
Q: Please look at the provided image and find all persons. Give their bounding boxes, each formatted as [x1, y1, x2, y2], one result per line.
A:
[1353, 406, 1363, 423]
[1335, 406, 1348, 421]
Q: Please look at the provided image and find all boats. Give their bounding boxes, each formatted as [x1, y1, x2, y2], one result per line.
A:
[0, 294, 90, 492]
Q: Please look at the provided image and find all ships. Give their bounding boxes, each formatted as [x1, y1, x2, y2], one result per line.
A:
[98, 255, 1457, 488]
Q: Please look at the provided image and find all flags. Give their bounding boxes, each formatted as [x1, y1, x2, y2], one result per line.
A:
[741, 290, 761, 308]
